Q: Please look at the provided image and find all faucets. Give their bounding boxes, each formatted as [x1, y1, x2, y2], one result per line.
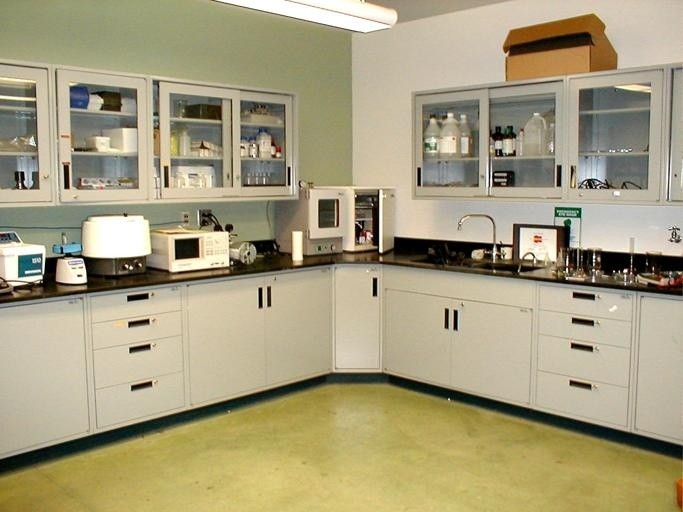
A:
[457, 213, 498, 263]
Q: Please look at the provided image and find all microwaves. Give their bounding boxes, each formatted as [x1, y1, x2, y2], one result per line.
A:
[149, 230, 229, 273]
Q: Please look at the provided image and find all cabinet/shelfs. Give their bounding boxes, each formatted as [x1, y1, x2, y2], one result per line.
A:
[187, 268, 330, 407]
[535, 282, 635, 435]
[342, 186, 395, 254]
[152, 75, 298, 202]
[0, 297, 89, 457]
[664, 62, 683, 206]
[0, 60, 55, 208]
[380, 263, 535, 412]
[633, 290, 683, 445]
[55, 64, 151, 204]
[413, 77, 565, 202]
[332, 264, 380, 372]
[89, 284, 186, 432]
[564, 66, 664, 206]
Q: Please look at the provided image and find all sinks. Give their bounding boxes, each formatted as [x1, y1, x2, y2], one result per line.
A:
[411, 256, 544, 273]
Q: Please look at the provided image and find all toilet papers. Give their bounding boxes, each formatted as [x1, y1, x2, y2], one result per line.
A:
[292, 230, 304, 262]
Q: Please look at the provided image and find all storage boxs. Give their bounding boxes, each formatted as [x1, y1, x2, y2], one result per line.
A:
[503, 13, 618, 81]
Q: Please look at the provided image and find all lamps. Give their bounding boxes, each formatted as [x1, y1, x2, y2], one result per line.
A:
[212, 0, 398, 33]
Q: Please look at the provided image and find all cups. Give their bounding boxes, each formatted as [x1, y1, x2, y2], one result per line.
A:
[501, 246, 513, 260]
[244, 171, 270, 185]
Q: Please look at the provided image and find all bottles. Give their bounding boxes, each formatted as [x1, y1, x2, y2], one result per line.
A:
[440, 111, 460, 159]
[240, 136, 248, 157]
[490, 125, 523, 157]
[180, 131, 191, 157]
[249, 135, 258, 158]
[556, 248, 601, 272]
[257, 128, 272, 158]
[458, 113, 473, 157]
[422, 117, 440, 157]
